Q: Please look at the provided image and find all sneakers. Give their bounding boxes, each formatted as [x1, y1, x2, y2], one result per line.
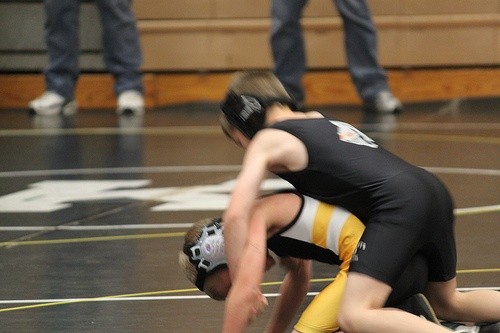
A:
[373, 91, 403, 113]
[28, 90, 66, 115]
[117, 88, 142, 114]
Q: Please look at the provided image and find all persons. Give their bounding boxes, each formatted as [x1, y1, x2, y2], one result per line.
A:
[269, 0, 402, 114]
[29, 0, 145, 116]
[218, 70, 500, 333]
[178, 189, 441, 333]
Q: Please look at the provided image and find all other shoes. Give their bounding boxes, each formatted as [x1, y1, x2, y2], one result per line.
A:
[400, 293, 440, 325]
